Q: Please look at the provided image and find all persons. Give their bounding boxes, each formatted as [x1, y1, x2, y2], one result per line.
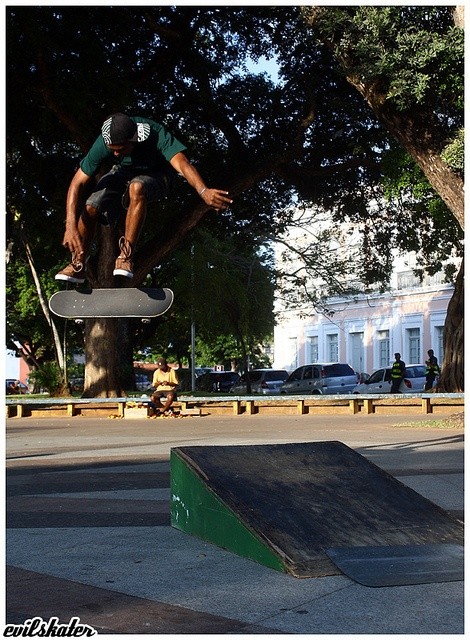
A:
[53, 112, 234, 284]
[424, 349, 440, 392]
[10, 379, 22, 394]
[149, 358, 181, 419]
[387, 352, 408, 394]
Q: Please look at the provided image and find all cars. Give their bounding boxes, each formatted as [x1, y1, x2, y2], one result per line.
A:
[352, 364, 441, 394]
[135, 367, 214, 396]
[229, 369, 290, 397]
[6, 379, 28, 395]
[280, 363, 360, 395]
[195, 373, 241, 394]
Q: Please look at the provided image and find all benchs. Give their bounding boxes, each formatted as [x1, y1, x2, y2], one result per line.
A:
[356, 392, 464, 414]
[127, 395, 238, 416]
[6, 400, 14, 417]
[239, 394, 356, 415]
[15, 398, 127, 417]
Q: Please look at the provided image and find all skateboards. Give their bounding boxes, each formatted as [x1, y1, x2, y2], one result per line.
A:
[48, 287, 174, 325]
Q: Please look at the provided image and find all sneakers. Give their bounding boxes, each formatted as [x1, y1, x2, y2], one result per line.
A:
[54, 250, 87, 284]
[168, 409, 175, 416]
[113, 236, 136, 279]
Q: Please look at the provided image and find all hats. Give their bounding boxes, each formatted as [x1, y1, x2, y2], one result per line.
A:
[156, 357, 168, 369]
[428, 350, 434, 354]
[395, 353, 401, 358]
[101, 112, 151, 146]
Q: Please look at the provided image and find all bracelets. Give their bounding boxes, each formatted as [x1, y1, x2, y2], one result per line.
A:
[199, 186, 208, 196]
